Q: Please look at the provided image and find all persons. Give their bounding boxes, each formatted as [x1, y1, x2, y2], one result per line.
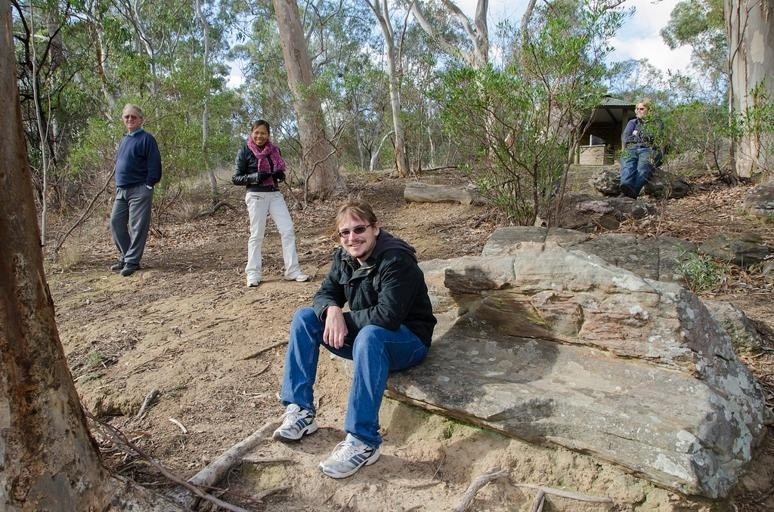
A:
[273, 200, 438, 480]
[230, 120, 311, 288]
[619, 98, 666, 200]
[109, 103, 162, 277]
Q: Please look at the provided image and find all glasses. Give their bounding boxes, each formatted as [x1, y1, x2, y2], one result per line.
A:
[637, 107, 647, 110]
[338, 224, 374, 239]
[124, 114, 137, 121]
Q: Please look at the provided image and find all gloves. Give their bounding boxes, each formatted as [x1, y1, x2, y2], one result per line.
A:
[258, 171, 272, 183]
[273, 170, 284, 181]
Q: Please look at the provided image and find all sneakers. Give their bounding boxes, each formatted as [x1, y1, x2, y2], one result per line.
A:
[247, 278, 261, 287]
[618, 184, 637, 199]
[121, 263, 138, 276]
[319, 431, 380, 479]
[273, 403, 318, 442]
[110, 261, 123, 269]
[293, 274, 311, 282]
